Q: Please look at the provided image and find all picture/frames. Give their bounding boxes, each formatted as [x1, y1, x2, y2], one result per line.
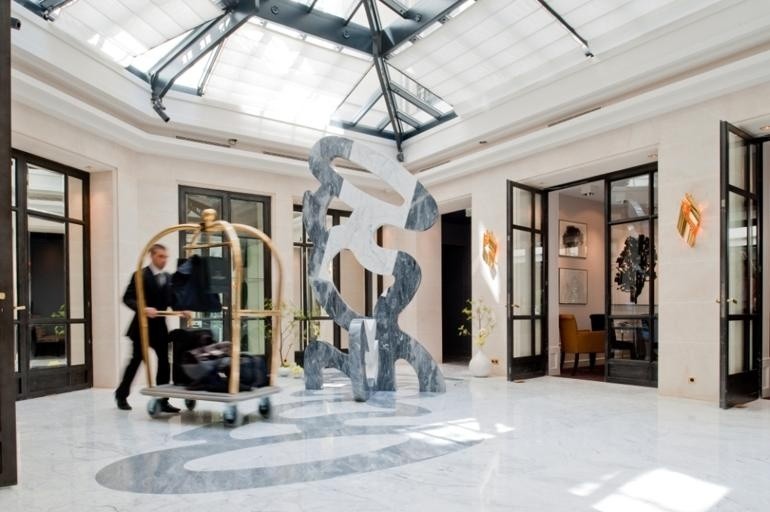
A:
[558, 268, 589, 306]
[558, 218, 589, 258]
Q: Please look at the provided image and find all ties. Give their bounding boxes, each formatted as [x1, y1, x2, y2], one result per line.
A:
[156, 272, 163, 286]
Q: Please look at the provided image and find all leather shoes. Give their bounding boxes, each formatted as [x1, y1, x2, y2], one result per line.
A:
[157, 398, 181, 413]
[115, 390, 132, 411]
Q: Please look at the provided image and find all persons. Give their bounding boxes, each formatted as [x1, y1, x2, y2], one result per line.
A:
[114, 243, 181, 415]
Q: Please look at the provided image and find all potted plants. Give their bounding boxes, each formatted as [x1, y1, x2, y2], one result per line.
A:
[262, 297, 320, 377]
[458, 296, 519, 379]
[292, 366, 303, 379]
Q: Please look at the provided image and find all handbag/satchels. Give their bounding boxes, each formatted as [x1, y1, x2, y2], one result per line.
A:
[181, 341, 267, 394]
[167, 253, 224, 311]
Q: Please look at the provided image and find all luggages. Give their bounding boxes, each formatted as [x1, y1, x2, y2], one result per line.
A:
[169, 325, 219, 385]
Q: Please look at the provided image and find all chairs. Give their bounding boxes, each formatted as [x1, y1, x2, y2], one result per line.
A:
[33, 312, 65, 360]
[559, 313, 658, 378]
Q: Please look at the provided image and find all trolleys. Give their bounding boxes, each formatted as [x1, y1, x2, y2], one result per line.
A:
[135, 208, 282, 426]
